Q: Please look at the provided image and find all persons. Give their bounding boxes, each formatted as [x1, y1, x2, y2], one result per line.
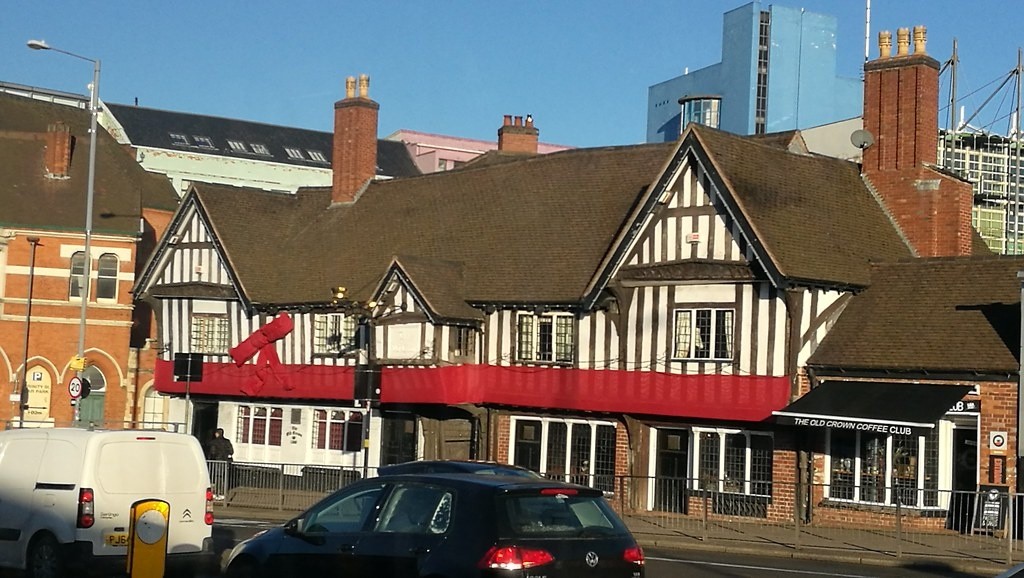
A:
[210, 428, 234, 476]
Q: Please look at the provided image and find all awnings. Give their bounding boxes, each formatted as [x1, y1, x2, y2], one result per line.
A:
[772, 380, 981, 438]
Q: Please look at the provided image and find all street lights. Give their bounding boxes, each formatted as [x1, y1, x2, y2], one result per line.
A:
[28, 36, 100, 425]
[20, 237, 46, 425]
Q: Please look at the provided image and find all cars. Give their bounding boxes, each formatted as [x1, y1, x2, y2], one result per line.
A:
[215, 460, 646, 578]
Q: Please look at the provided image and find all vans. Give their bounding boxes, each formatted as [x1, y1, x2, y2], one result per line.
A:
[0, 428, 214, 577]
